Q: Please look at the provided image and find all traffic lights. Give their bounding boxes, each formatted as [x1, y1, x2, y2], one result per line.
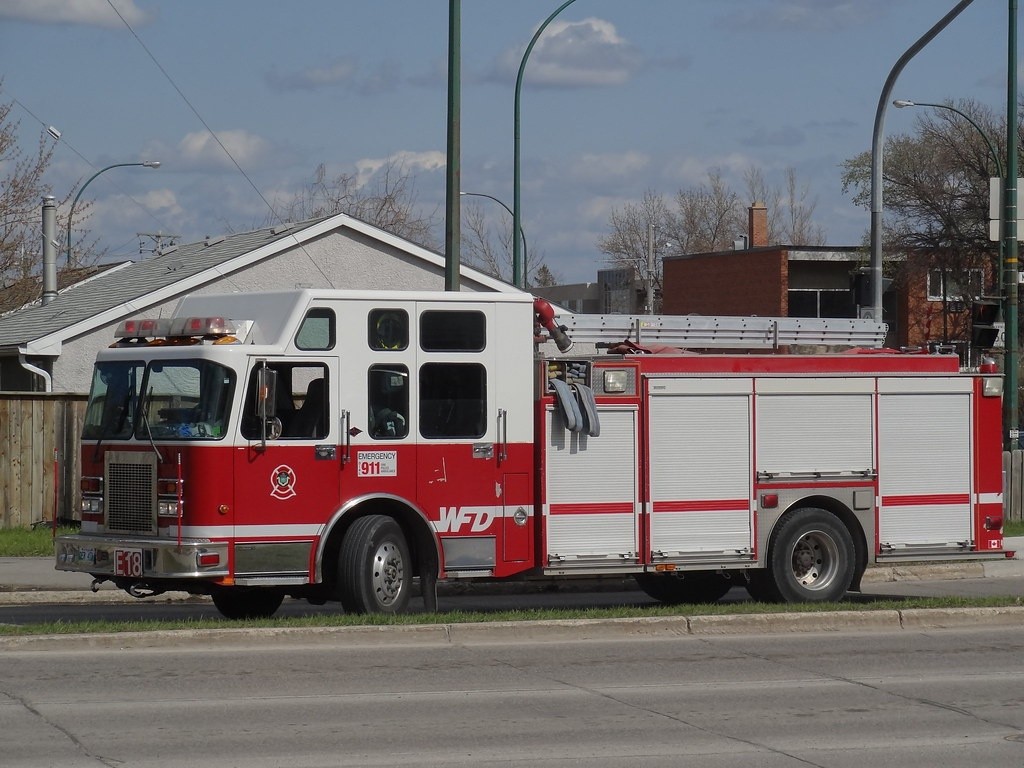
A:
[970, 301, 1000, 349]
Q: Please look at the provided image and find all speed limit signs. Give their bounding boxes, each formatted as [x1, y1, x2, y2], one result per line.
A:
[992, 322, 1006, 349]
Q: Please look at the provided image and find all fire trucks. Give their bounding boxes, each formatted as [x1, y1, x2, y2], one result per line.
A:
[52, 288, 1017, 621]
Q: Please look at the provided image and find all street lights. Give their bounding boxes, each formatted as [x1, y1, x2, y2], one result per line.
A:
[893, 100, 1002, 321]
[461, 192, 528, 290]
[67, 162, 162, 269]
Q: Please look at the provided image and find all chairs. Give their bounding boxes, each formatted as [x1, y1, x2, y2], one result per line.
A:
[285, 378, 323, 437]
